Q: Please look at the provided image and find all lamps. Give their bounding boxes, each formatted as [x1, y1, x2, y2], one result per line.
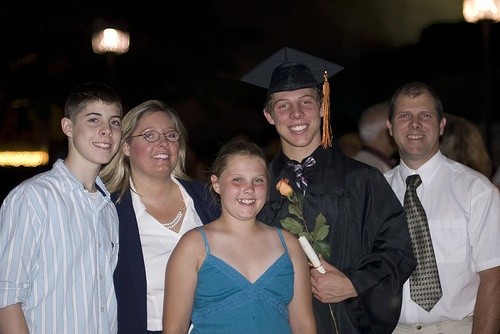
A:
[91, 15, 131, 66]
[462, 0, 500, 40]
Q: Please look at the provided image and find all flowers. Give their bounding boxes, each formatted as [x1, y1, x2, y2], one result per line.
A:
[275, 177, 339, 334]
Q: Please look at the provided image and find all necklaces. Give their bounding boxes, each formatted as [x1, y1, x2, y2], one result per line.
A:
[163, 199, 184, 228]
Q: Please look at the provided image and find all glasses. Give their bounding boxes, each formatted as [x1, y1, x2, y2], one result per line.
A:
[128, 130, 181, 142]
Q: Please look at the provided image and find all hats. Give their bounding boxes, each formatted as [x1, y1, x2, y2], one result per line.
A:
[240, 46, 345, 150]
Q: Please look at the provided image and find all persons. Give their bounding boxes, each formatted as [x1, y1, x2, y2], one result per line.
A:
[99, 100, 221, 334]
[0, 80, 123, 334]
[161, 141, 320, 334]
[239, 47, 500, 334]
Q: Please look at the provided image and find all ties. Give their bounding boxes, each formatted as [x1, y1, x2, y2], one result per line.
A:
[286, 157, 315, 197]
[403, 174, 443, 313]
